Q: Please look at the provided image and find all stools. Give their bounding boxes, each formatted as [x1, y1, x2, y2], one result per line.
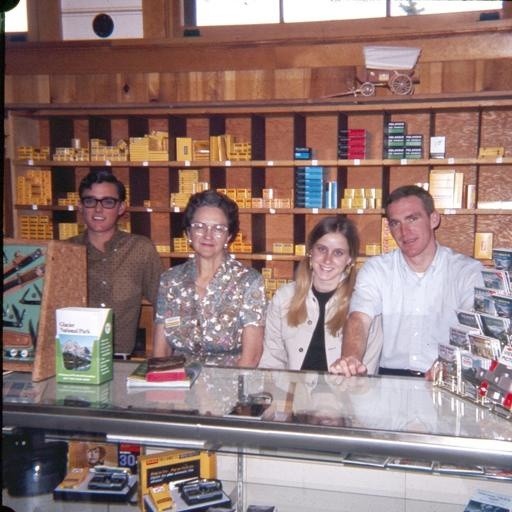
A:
[376, 367, 426, 378]
[110, 354, 133, 361]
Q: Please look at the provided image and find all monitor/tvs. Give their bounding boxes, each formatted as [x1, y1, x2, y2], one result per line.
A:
[126, 359, 201, 388]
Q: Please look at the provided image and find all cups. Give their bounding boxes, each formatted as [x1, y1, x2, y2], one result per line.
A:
[79, 195, 122, 209]
[185, 221, 229, 241]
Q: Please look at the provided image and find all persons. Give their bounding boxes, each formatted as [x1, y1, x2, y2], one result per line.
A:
[152, 189, 268, 368]
[256, 216, 384, 376]
[272, 371, 350, 427]
[330, 184, 483, 381]
[62, 169, 167, 361]
[336, 375, 438, 434]
[86, 446, 106, 468]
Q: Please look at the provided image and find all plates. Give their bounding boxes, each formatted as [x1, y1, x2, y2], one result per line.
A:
[2, 90, 512, 359]
